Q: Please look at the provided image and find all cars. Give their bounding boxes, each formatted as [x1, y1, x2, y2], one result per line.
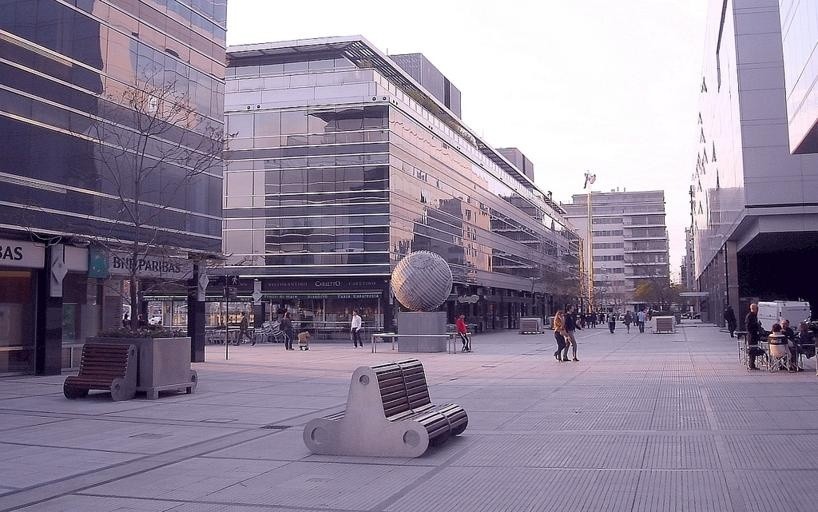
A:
[617, 312, 627, 321]
[149, 316, 162, 326]
[680, 311, 697, 318]
[694, 313, 701, 319]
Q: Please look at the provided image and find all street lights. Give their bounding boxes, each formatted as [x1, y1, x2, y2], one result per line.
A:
[584, 170, 595, 314]
[576, 296, 589, 328]
[600, 289, 606, 309]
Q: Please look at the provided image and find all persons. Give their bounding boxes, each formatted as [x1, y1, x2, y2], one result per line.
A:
[232, 311, 256, 346]
[723, 305, 737, 337]
[579, 307, 652, 334]
[553, 311, 566, 361]
[744, 304, 817, 372]
[563, 304, 584, 361]
[298, 330, 311, 350]
[456, 313, 471, 352]
[283, 311, 294, 350]
[350, 310, 363, 349]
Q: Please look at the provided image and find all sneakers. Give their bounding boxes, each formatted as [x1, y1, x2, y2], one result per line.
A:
[747, 365, 760, 371]
[353, 345, 364, 348]
[462, 347, 471, 352]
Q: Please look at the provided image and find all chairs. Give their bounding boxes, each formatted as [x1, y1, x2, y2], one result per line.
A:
[734, 330, 818, 377]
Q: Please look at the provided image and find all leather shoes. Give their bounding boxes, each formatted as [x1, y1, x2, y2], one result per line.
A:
[553, 351, 580, 361]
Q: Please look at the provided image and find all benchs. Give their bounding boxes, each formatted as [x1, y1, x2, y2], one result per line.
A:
[302, 358, 469, 458]
[63, 342, 138, 402]
[655, 317, 673, 334]
[191, 369, 198, 389]
[518, 320, 539, 335]
